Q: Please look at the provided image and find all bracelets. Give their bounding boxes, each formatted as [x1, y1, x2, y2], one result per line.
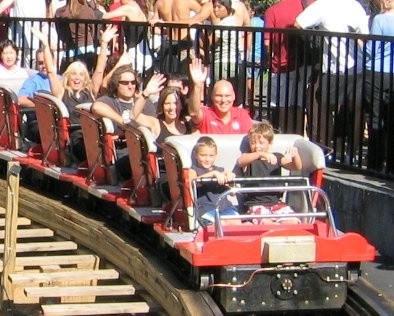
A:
[43, 43, 49, 49]
[100, 46, 108, 50]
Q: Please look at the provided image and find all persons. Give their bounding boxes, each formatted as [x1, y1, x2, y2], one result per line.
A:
[189, 137, 242, 230]
[237, 120, 304, 224]
[0, 0, 394, 210]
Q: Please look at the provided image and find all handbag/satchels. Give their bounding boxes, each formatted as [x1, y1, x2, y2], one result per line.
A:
[284, 26, 323, 66]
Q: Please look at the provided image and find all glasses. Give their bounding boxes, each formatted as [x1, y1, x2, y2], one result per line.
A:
[37, 61, 43, 65]
[118, 80, 137, 85]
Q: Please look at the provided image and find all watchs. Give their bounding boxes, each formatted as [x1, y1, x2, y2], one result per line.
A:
[142, 94, 149, 101]
